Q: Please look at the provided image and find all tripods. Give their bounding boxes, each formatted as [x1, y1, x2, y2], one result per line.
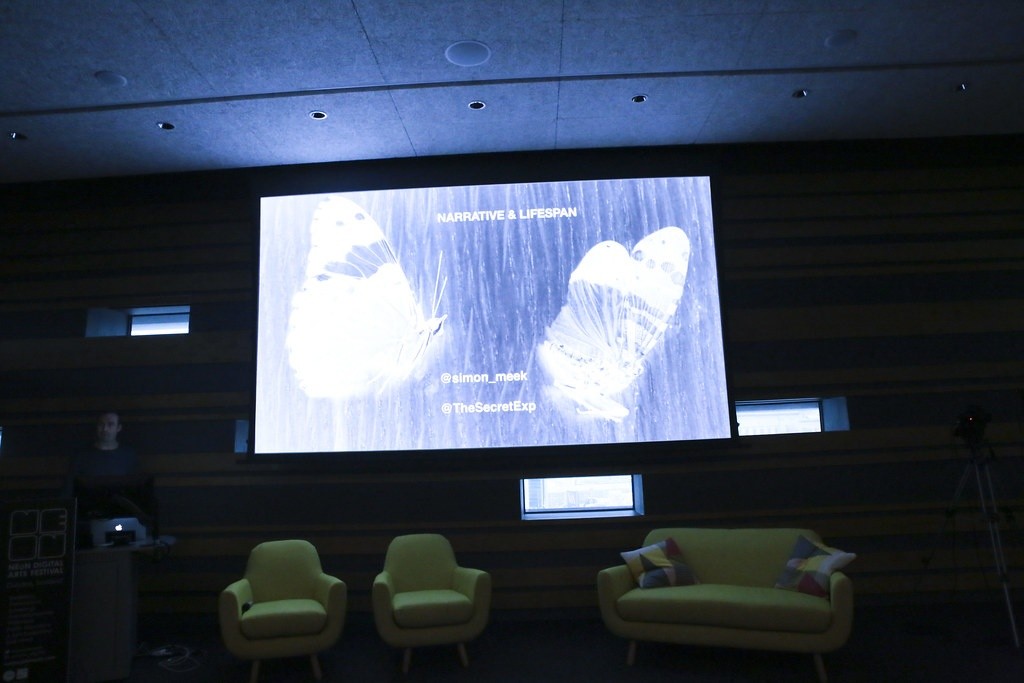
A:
[892, 445, 1022, 649]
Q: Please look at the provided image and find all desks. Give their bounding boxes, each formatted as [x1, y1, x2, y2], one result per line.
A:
[72, 546, 168, 683]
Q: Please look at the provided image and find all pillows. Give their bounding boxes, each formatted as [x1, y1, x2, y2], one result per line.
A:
[621, 537, 704, 590]
[773, 533, 857, 601]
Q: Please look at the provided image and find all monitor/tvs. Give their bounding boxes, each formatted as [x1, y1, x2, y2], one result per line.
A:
[73, 474, 154, 547]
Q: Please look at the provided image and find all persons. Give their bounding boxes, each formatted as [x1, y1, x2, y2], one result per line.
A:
[73, 410, 158, 549]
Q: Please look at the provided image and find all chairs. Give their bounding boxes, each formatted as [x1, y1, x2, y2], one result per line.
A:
[371, 533, 494, 676]
[219, 539, 348, 682]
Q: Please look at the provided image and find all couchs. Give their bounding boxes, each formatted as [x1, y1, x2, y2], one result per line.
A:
[597, 528, 856, 682]
